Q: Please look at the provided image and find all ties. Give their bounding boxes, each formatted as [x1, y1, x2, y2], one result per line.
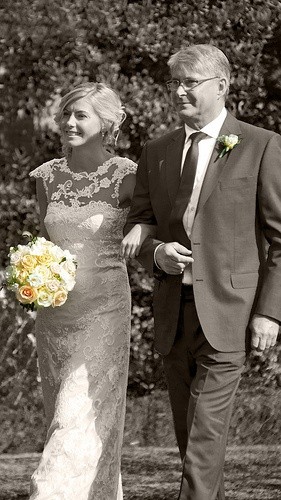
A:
[161, 131, 208, 242]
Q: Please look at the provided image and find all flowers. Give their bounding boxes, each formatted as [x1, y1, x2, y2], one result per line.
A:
[216, 134, 245, 158]
[0, 231, 78, 313]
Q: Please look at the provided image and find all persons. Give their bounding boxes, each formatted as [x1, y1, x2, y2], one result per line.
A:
[121, 45, 281, 500]
[28, 80, 158, 500]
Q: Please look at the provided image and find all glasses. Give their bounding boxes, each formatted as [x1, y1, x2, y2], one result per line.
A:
[166, 76, 220, 90]
